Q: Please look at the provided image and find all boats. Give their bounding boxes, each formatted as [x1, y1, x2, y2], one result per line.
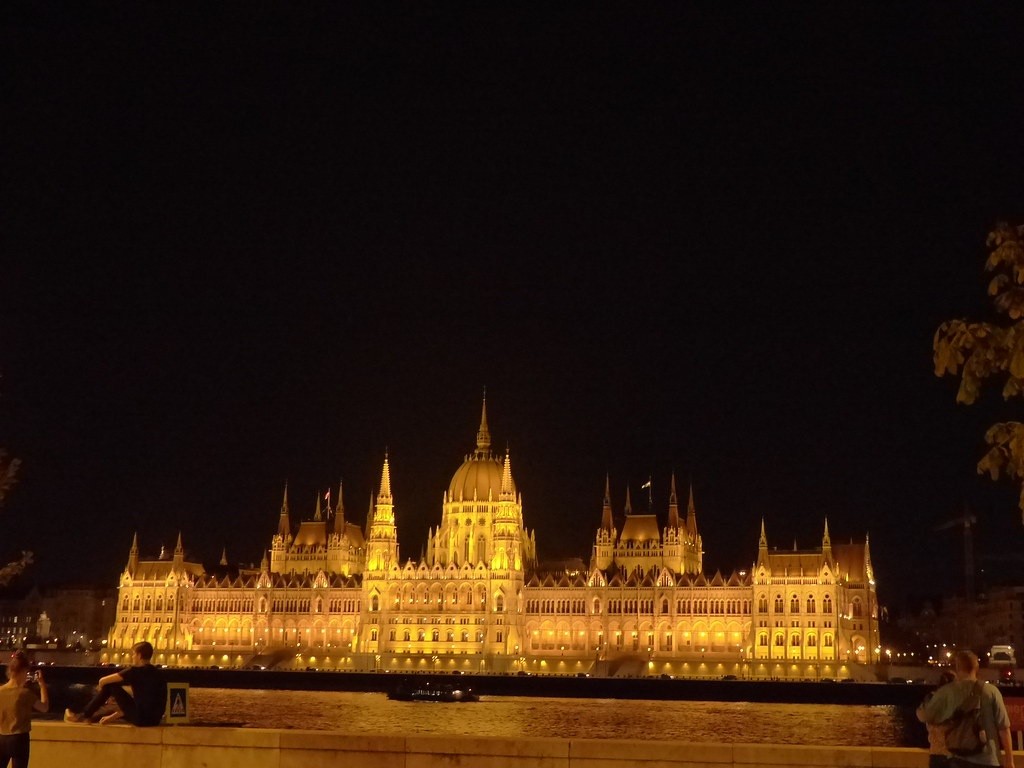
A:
[384, 677, 481, 703]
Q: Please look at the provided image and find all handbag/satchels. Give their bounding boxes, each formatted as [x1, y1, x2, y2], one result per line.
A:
[944, 695, 987, 756]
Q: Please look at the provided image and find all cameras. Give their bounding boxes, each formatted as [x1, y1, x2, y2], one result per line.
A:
[27, 672, 39, 682]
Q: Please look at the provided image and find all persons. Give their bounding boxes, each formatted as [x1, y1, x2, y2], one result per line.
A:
[64, 643, 167, 726]
[916, 649, 1015, 768]
[0, 650, 49, 768]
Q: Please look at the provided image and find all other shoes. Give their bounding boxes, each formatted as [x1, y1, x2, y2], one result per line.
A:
[63, 708, 91, 724]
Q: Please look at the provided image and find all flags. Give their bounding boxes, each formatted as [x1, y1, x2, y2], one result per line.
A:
[325, 492, 329, 499]
